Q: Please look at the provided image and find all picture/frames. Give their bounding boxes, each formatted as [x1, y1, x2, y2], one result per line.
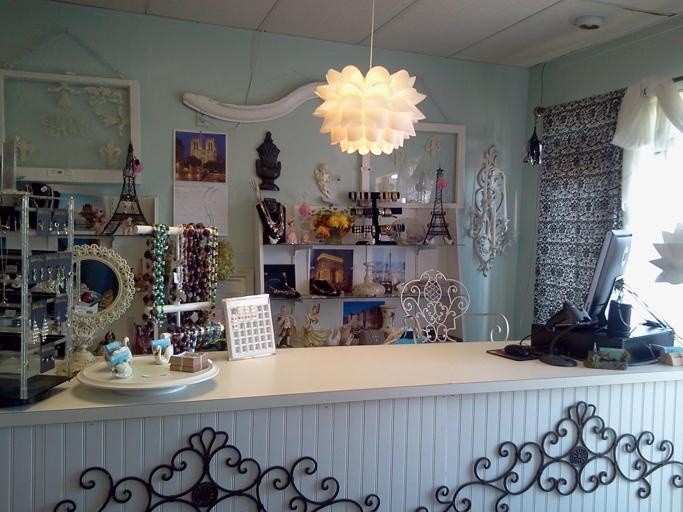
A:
[0, 69, 141, 185]
[362, 122, 466, 209]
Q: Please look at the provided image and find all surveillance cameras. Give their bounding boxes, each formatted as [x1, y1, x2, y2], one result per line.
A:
[575, 15, 602, 31]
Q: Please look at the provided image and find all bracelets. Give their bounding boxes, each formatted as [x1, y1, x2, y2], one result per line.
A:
[173, 322, 223, 355]
[351, 190, 398, 219]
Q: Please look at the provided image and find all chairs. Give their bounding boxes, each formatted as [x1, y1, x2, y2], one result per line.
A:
[400, 270, 509, 343]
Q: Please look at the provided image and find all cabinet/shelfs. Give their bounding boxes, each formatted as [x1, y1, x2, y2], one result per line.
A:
[0, 195, 74, 399]
[255, 204, 465, 342]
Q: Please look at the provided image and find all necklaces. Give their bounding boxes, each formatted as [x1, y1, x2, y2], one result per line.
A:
[268, 280, 297, 297]
[31, 185, 54, 210]
[259, 200, 285, 237]
[313, 279, 337, 294]
[143, 222, 218, 331]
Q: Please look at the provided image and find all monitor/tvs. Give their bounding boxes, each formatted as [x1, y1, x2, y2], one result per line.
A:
[583, 230, 638, 337]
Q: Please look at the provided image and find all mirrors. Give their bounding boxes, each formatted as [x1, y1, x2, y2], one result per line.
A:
[73, 243, 135, 371]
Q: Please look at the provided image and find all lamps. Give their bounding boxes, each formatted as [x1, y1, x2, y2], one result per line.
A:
[313, 0, 427, 155]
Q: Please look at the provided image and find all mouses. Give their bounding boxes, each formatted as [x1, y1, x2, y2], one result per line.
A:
[504, 344, 530, 357]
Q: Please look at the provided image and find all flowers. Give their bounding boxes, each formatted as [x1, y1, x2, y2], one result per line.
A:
[308, 210, 353, 242]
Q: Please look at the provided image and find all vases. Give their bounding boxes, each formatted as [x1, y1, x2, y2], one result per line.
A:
[353, 262, 386, 298]
[325, 231, 343, 244]
